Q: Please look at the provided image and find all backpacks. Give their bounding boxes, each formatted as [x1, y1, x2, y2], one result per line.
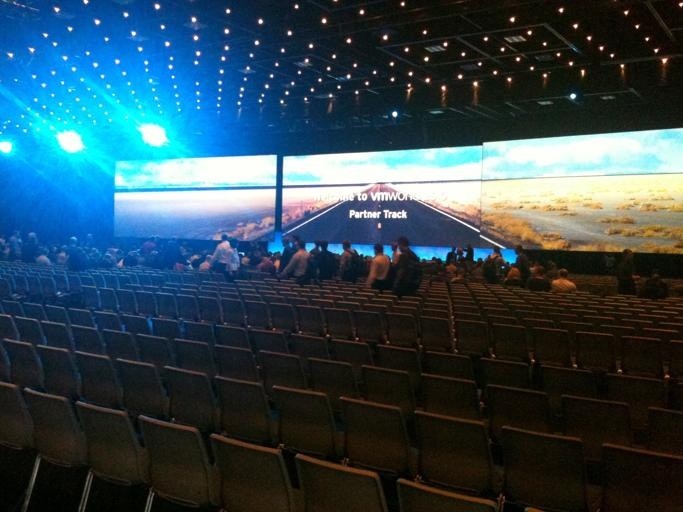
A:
[483, 255, 498, 278]
[346, 249, 363, 275]
[297, 251, 320, 280]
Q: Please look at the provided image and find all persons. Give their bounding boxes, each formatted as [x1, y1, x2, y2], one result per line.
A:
[619, 249, 636, 295]
[1, 230, 168, 271]
[640, 269, 669, 298]
[167, 231, 337, 280]
[421, 243, 474, 282]
[473, 244, 577, 293]
[339, 236, 416, 290]
